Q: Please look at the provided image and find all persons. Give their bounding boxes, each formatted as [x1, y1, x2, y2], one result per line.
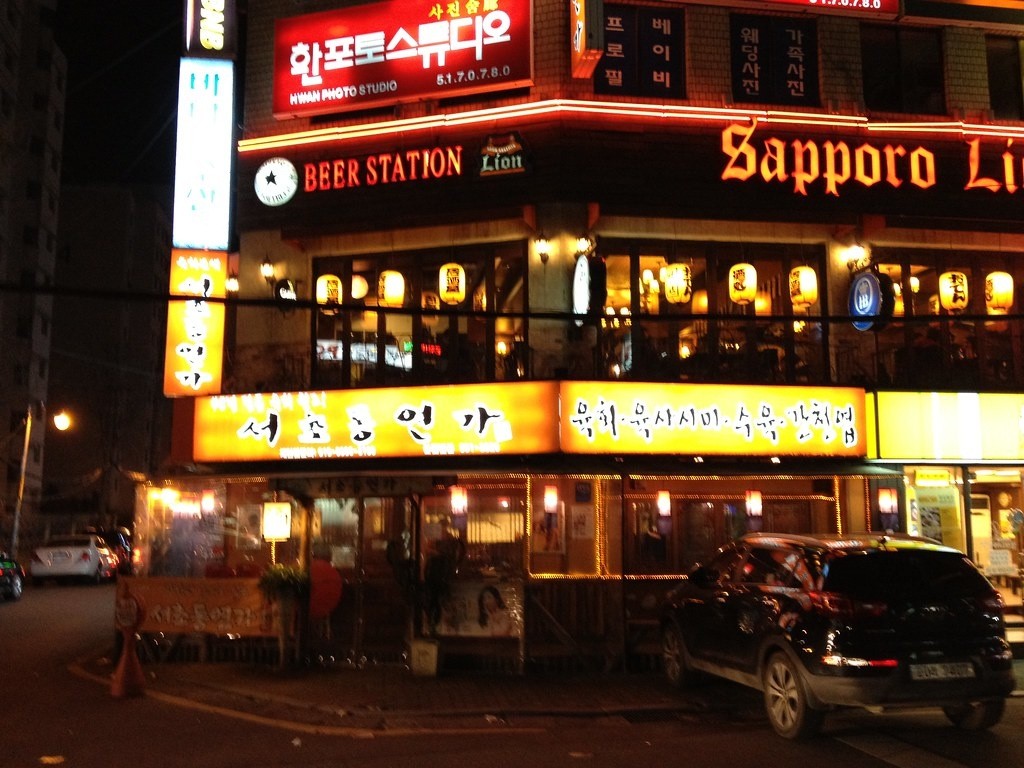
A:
[475, 585, 514, 637]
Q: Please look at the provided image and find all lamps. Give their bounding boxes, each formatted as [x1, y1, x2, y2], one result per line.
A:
[837, 243, 869, 269]
[315, 272, 342, 315]
[573, 232, 592, 260]
[865, 478, 875, 491]
[376, 268, 404, 310]
[983, 271, 1014, 312]
[729, 256, 759, 307]
[199, 482, 217, 517]
[664, 256, 692, 306]
[225, 266, 238, 294]
[495, 340, 510, 359]
[260, 478, 294, 544]
[744, 480, 763, 518]
[439, 261, 467, 305]
[449, 476, 470, 517]
[532, 230, 552, 262]
[542, 477, 561, 517]
[937, 271, 969, 314]
[260, 254, 276, 286]
[909, 276, 922, 292]
[656, 480, 672, 518]
[637, 260, 667, 294]
[692, 289, 710, 315]
[788, 259, 819, 310]
[349, 274, 371, 300]
[601, 301, 634, 328]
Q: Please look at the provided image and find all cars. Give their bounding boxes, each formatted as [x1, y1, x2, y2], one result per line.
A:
[30, 533, 119, 586]
[0, 544, 26, 600]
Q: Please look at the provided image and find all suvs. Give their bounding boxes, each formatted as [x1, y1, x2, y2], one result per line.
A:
[659, 533, 1018, 740]
